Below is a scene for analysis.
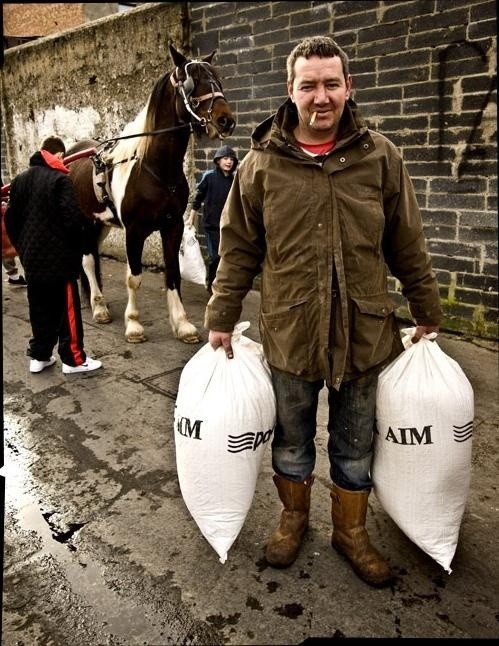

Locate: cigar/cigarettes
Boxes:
[309,111,317,126]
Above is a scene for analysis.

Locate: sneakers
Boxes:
[6,274,28,291]
[61,356,103,374]
[28,354,57,374]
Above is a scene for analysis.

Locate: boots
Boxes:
[264,472,317,569]
[329,478,395,590]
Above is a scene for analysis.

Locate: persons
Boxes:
[202,35,445,589]
[192,144,238,297]
[4,135,103,374]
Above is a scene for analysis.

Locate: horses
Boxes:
[62,42,238,346]
